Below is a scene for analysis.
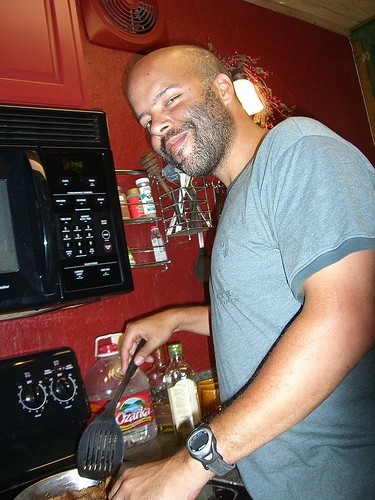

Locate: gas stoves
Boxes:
[0,346,92,500]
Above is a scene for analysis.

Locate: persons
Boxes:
[107,44,375,500]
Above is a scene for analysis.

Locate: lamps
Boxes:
[230,70,263,116]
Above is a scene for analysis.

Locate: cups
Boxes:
[197,377,221,418]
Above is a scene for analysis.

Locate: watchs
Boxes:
[185,422,236,477]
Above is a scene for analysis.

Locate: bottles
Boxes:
[135,178,157,215]
[126,188,145,219]
[117,186,131,221]
[84,332,158,449]
[165,344,204,440]
[151,226,168,262]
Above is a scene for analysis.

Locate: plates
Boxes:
[12,461,144,500]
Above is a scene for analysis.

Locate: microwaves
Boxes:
[0,102,135,321]
